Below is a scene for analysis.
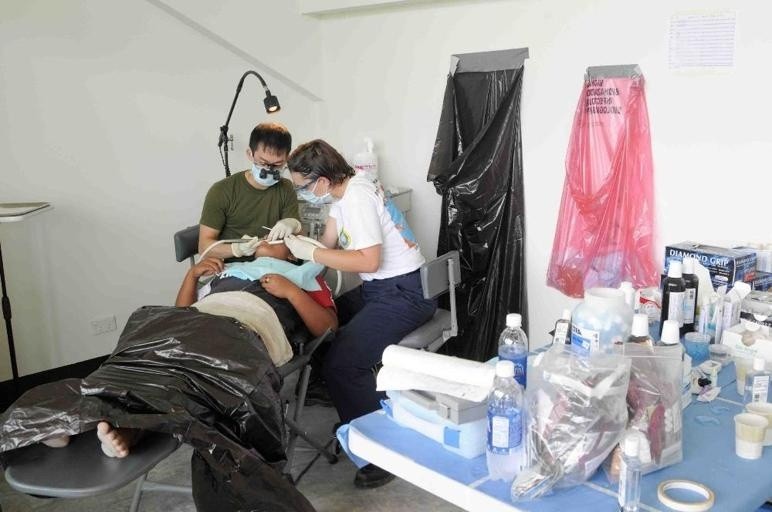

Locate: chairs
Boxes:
[170,220,214,292]
[6,235,343,512]
[393,250,462,354]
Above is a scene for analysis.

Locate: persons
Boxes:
[197,121,308,257]
[288,138,439,488]
[39,236,339,460]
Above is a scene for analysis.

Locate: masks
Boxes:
[300,177,332,206]
[250,157,286,187]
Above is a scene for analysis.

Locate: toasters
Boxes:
[552,310,571,344]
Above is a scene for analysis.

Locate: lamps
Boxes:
[220,68,281,176]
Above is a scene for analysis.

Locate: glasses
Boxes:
[252,154,286,172]
[293,178,319,194]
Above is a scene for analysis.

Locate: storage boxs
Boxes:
[664,242,756,284]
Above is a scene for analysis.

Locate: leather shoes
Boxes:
[353,463,395,489]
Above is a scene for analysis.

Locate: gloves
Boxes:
[284,234,316,265]
[268,218,302,241]
[231,234,262,258]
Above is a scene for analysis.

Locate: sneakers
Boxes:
[295,378,335,408]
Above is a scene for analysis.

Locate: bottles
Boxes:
[617,437,640,512]
[659,261,685,338]
[655,319,685,358]
[619,281,634,307]
[499,313,528,387]
[744,358,769,403]
[569,287,634,357]
[682,258,699,332]
[487,360,523,480]
[626,311,655,347]
[354,137,378,180]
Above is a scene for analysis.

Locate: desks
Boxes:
[0,202,50,387]
[343,287,772,511]
[297,187,412,240]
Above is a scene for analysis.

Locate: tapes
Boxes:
[657,478,714,512]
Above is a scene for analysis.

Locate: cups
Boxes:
[746,403,772,445]
[735,357,754,395]
[734,413,768,459]
[710,344,731,360]
[686,333,709,367]
